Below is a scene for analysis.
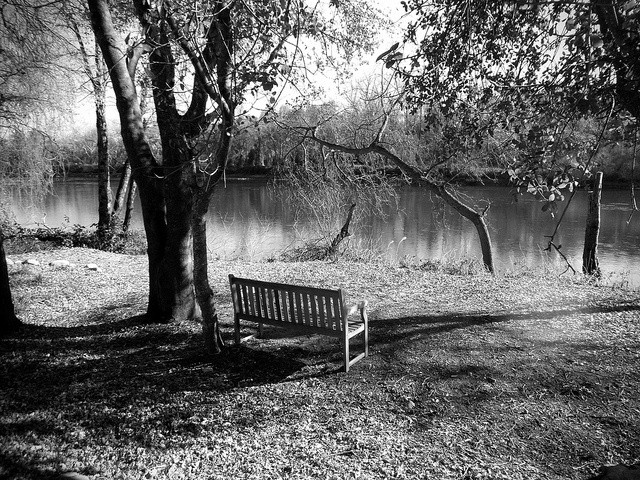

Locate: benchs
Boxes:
[226,273,370,373]
